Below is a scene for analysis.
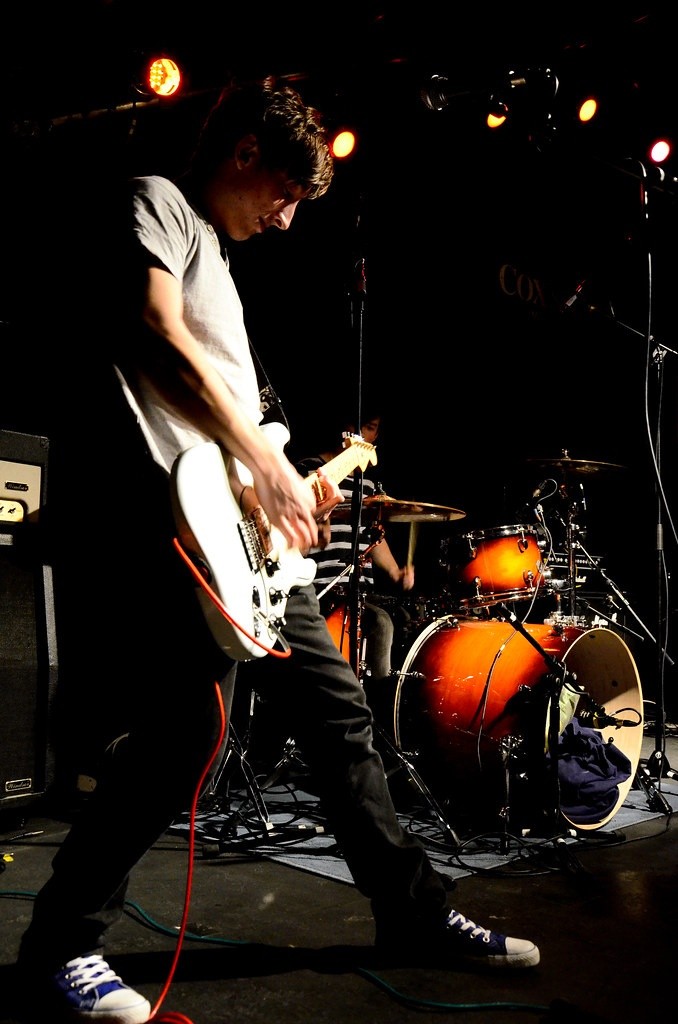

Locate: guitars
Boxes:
[167,419,381,665]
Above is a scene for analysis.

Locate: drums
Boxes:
[311,578,374,663]
[390,611,646,831]
[439,520,555,610]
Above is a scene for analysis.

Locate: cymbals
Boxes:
[346,493,469,528]
[525,456,619,474]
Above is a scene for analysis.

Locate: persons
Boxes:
[15,81,540,1024]
[298,401,415,679]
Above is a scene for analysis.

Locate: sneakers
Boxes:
[374,910,543,971]
[20,949,152,1024]
